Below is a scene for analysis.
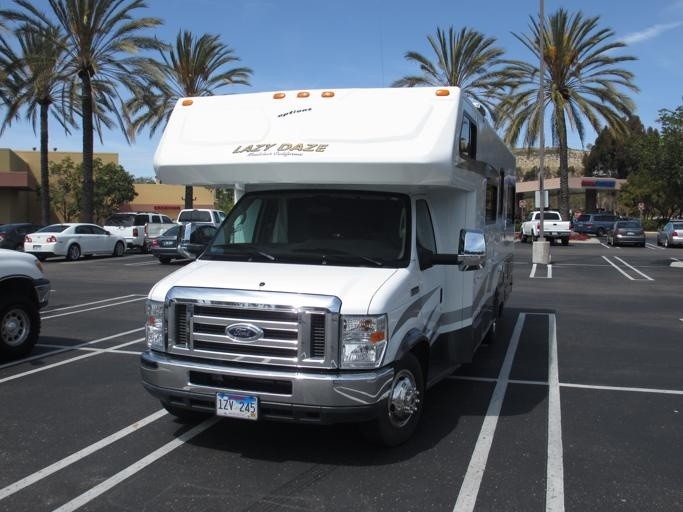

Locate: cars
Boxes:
[657,219,683,248]
[573,210,645,247]
[0,208,226,354]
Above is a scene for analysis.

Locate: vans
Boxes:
[140,86,517,443]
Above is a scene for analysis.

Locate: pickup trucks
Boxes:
[519,210,572,245]
